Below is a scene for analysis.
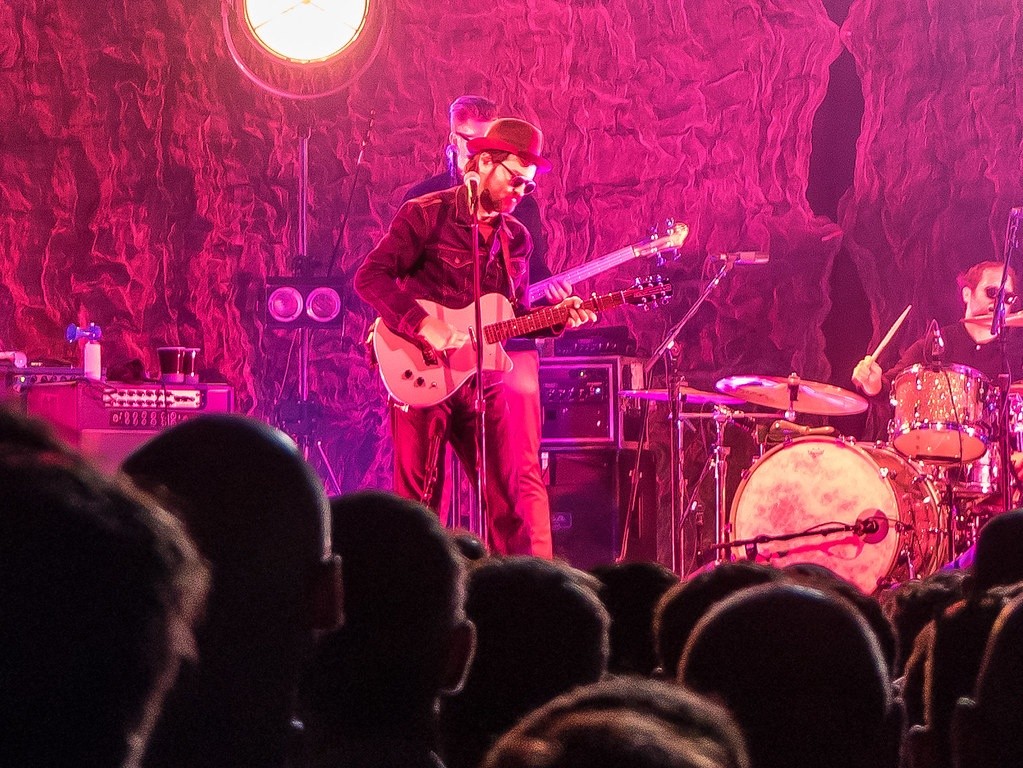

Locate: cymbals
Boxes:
[958,311,1023,327]
[716,373,869,416]
[619,388,748,404]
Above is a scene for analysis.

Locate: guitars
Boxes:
[371,274,676,407]
[528,216,691,304]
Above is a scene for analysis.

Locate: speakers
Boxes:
[533,447,656,573]
[21,380,234,478]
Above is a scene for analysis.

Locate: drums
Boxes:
[887,418,1005,499]
[730,434,950,599]
[891,360,995,464]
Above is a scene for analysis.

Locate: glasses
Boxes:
[493,159,536,195]
[984,287,1017,305]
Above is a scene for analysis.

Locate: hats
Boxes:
[466,118,553,175]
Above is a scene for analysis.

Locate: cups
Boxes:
[183,348,206,383]
[158,346,186,385]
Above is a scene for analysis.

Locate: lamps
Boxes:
[220,0,390,103]
[264,277,345,330]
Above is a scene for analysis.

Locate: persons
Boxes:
[353,119,597,559]
[0,402,1023,768]
[400,97,574,558]
[853,261,1023,404]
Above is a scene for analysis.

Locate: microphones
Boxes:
[852,520,880,534]
[464,171,481,213]
[710,402,756,431]
[932,323,941,373]
[712,251,770,264]
[1011,207,1023,218]
